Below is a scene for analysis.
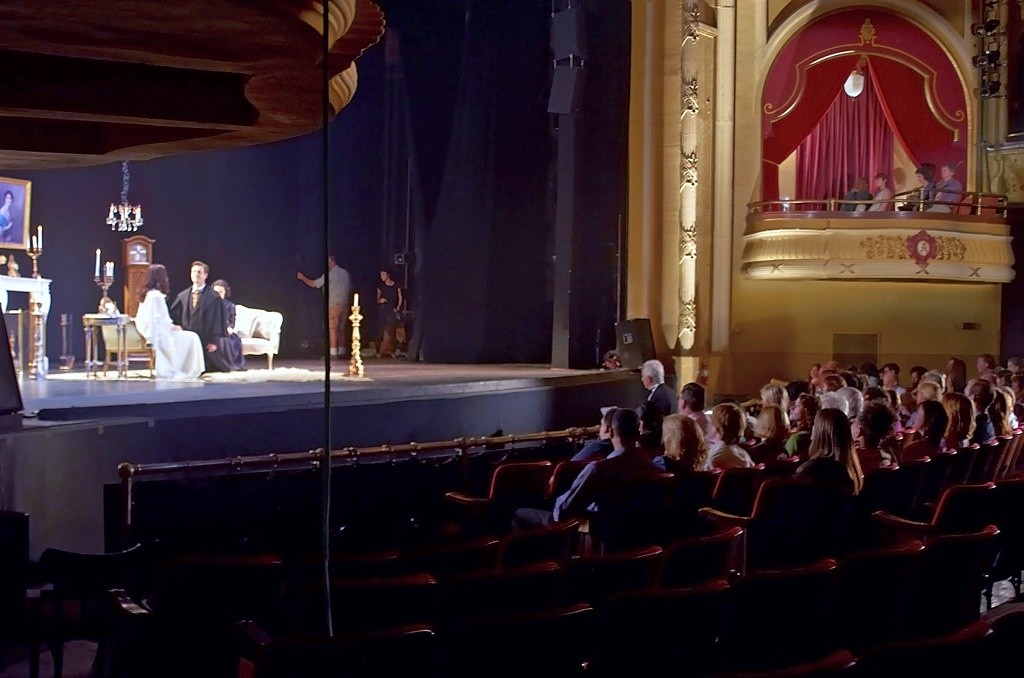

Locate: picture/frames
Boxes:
[0,176,32,249]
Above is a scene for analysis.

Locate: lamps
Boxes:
[843,70,865,102]
[26,225,43,278]
[94,248,114,314]
[971,18,1007,99]
[106,161,144,232]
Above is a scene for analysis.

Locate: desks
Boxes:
[1,275,53,380]
[83,313,130,379]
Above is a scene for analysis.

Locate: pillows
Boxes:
[233,305,259,338]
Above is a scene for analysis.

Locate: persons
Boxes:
[376,268,403,358]
[840,177,873,212]
[212,279,247,371]
[509,354,1024,529]
[297,254,352,360]
[915,167,935,199]
[135,265,206,378]
[927,163,962,212]
[169,261,230,372]
[867,173,891,211]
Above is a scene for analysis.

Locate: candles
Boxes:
[353,293,359,306]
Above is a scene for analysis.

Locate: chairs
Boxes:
[1,424,1024,677]
[102,317,156,379]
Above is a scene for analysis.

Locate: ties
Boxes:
[192,290,199,309]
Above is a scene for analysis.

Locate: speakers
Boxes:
[0,305,24,414]
[615,318,656,368]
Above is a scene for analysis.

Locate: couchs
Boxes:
[231,304,283,370]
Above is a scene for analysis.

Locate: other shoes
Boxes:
[321,354,338,360]
[338,354,346,359]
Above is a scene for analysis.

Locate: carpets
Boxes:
[46,367,375,384]
